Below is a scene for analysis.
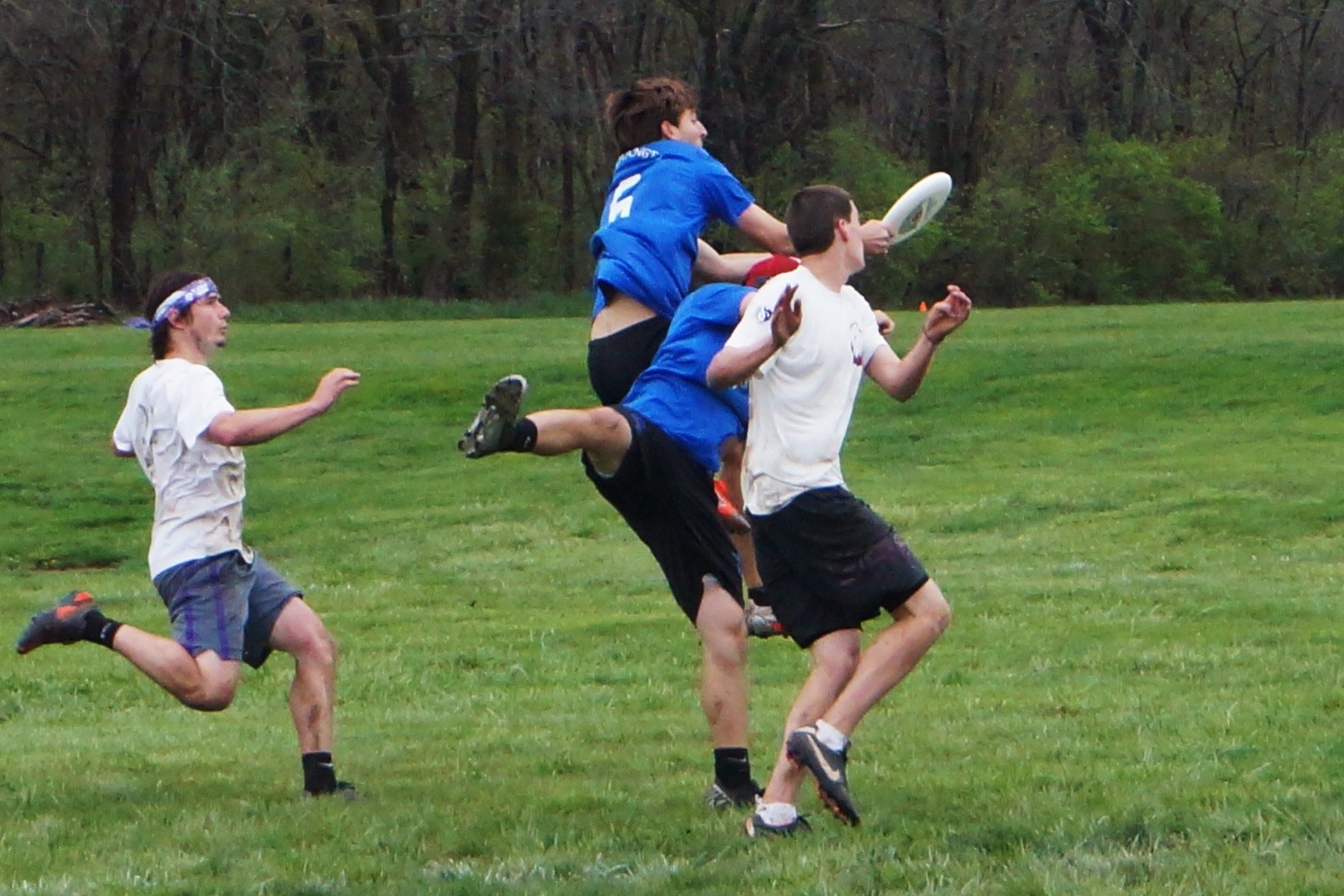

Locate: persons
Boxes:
[457,256,895,810]
[19,271,361,799]
[706,184,972,839]
[587,78,896,640]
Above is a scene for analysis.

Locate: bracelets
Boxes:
[922,325,943,343]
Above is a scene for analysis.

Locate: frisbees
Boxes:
[881,171,952,244]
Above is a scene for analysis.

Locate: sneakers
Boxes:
[711,780,764,814]
[713,484,755,535]
[14,589,99,655]
[304,782,365,800]
[742,598,790,638]
[743,812,809,836]
[458,373,527,460]
[786,725,861,827]
